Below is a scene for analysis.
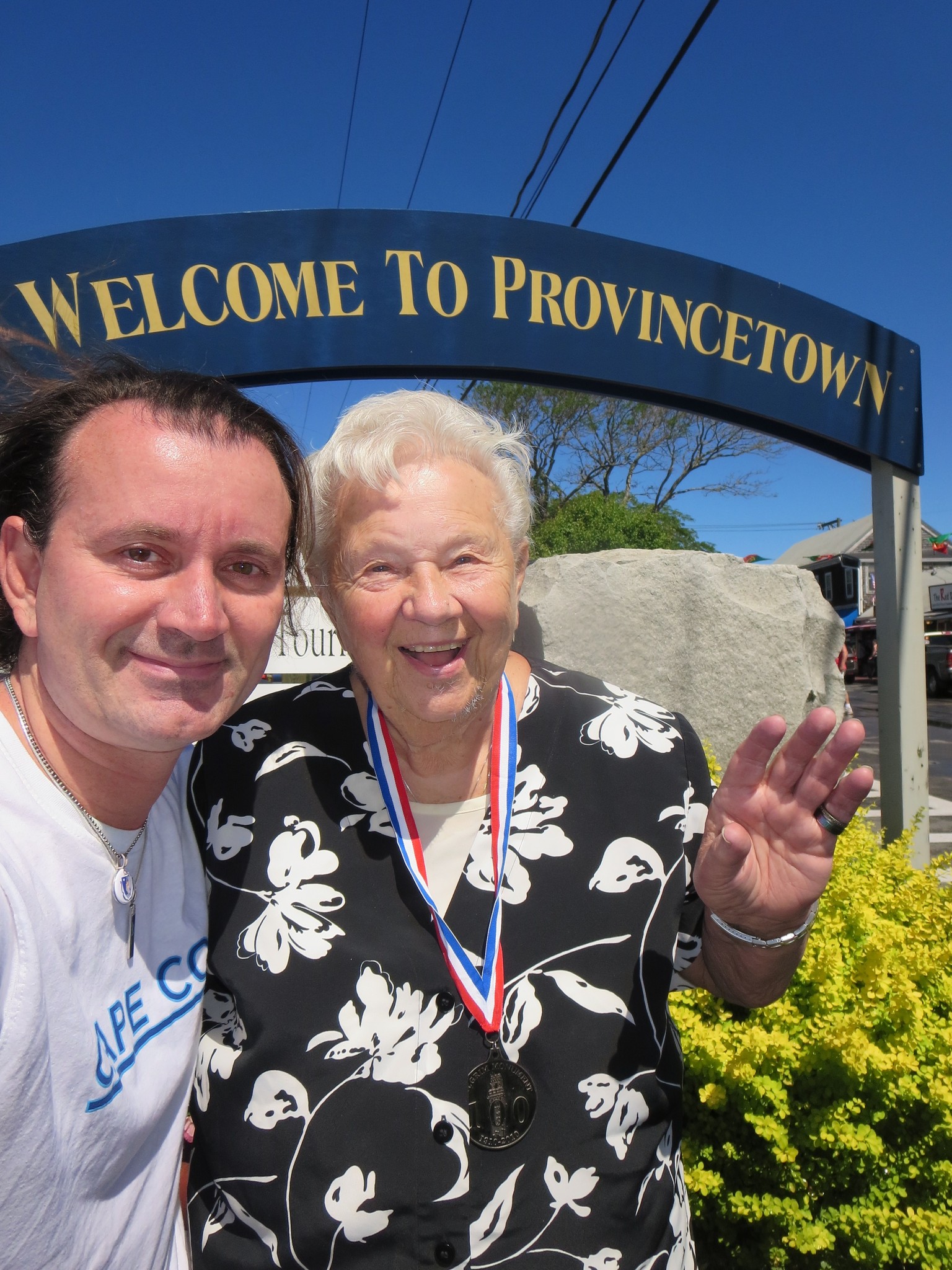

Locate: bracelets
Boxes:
[707,901,817,950]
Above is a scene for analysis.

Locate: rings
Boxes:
[816,804,847,836]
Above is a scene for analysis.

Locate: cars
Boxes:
[844,646,859,679]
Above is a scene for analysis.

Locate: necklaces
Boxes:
[4,673,150,959]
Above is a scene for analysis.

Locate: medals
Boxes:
[462,1056,539,1152]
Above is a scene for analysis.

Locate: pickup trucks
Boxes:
[925,645,952,695]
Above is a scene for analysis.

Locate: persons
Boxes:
[0,347,316,1269]
[183,390,876,1270]
[835,642,853,715]
[868,637,877,661]
